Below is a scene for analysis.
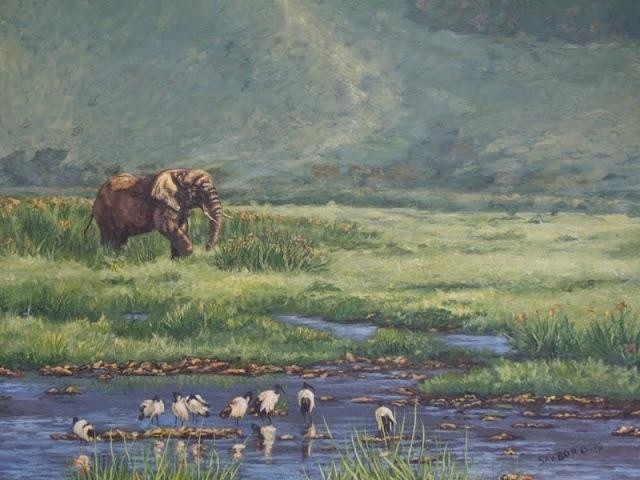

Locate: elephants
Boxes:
[82,167,235,262]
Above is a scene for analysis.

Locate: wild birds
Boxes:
[152,439,165,463]
[218,390,253,425]
[247,383,286,421]
[185,393,211,425]
[137,394,165,426]
[301,420,317,440]
[228,439,247,465]
[374,400,397,436]
[174,439,188,462]
[171,392,190,427]
[72,416,104,447]
[190,438,209,467]
[297,381,316,417]
[249,421,278,465]
[71,448,93,474]
